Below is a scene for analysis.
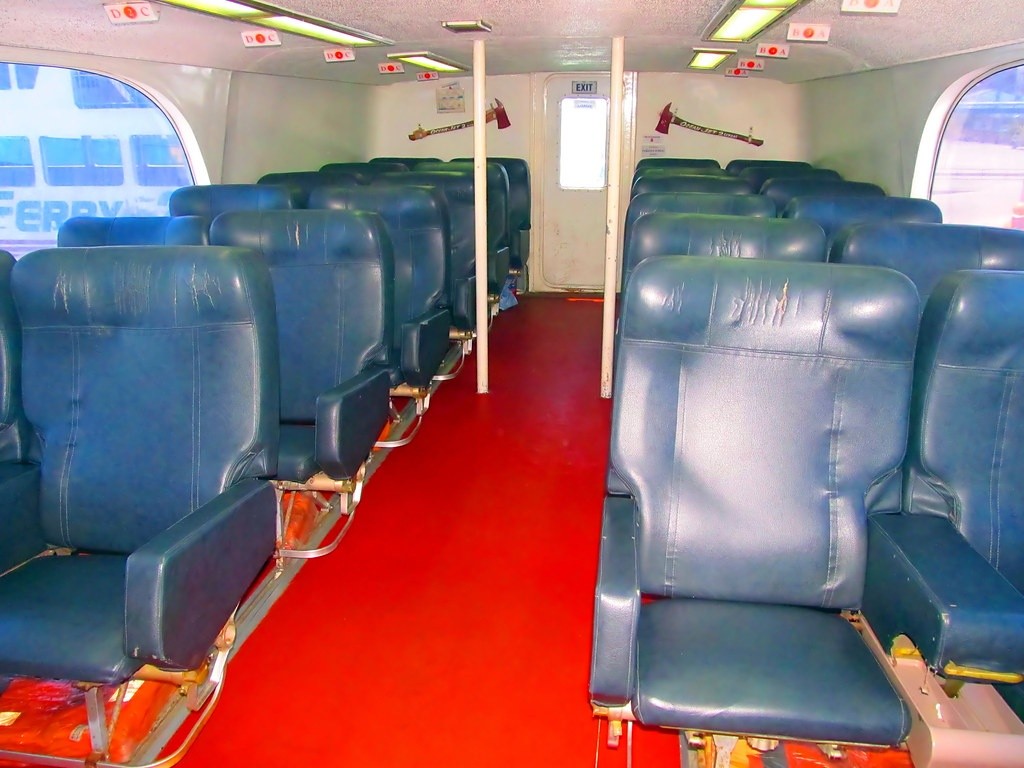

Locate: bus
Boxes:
[922,102,1023,229]
[1,63,194,260]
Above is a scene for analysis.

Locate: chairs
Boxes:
[0,158,531,768]
[590,158,1024,768]
